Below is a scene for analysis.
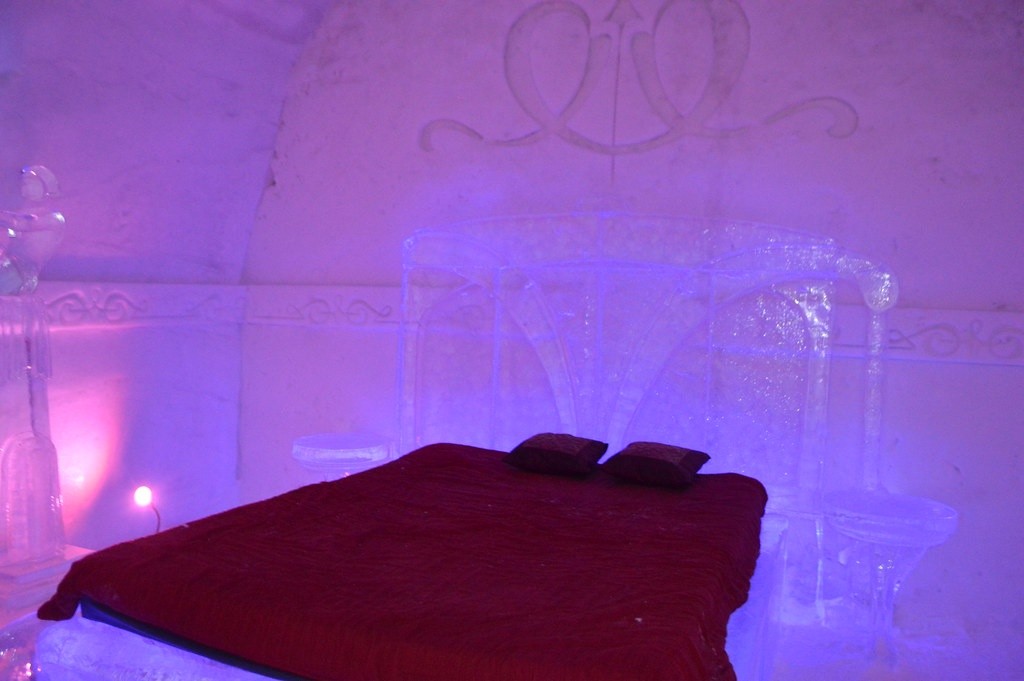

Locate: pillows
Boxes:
[502,432,610,480]
[600,441,710,491]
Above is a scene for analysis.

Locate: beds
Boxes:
[30,227,898,681]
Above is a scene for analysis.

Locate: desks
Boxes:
[820,493,956,664]
[292,433,388,481]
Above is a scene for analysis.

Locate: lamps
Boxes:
[131,485,162,535]
